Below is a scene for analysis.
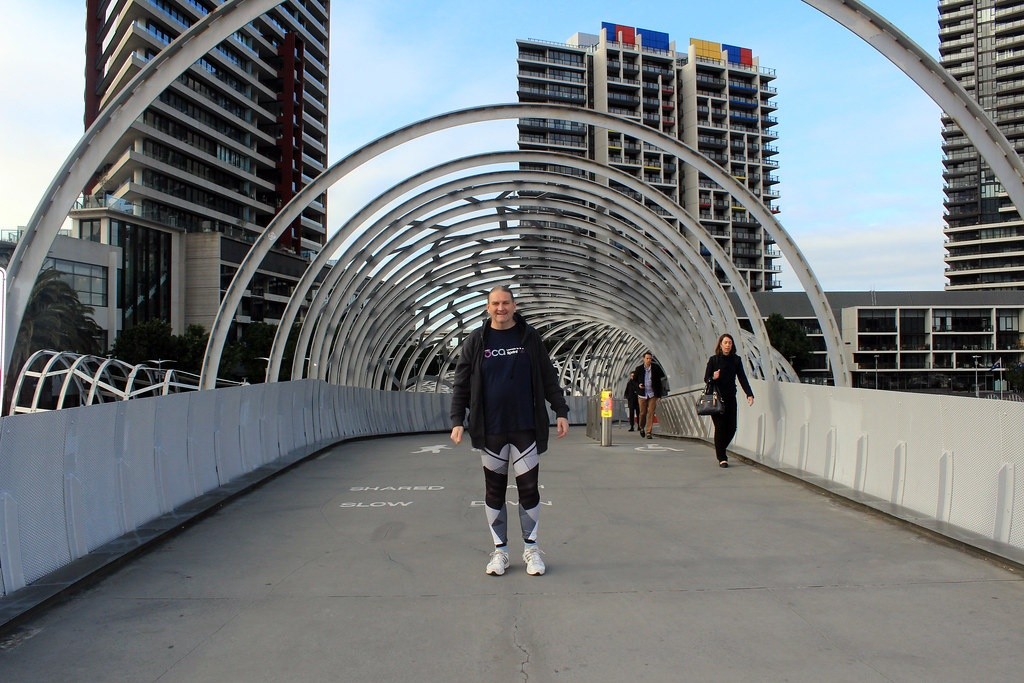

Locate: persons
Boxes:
[704,333,755,468]
[624,352,666,439]
[450,285,570,576]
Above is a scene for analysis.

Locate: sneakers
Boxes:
[719,458,728,468]
[646,432,652,439]
[486,549,510,576]
[628,428,634,431]
[522,545,546,576]
[640,428,645,437]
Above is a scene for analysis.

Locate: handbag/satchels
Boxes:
[696,375,724,415]
